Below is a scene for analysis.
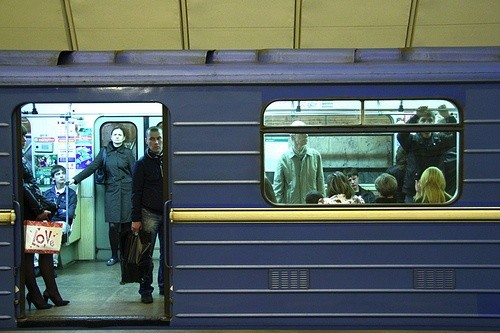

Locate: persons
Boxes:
[64,126,136,266]
[131,127,167,304]
[265,105,458,204]
[34,164,77,278]
[20,124,70,311]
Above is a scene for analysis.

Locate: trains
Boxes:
[0,46,500,331]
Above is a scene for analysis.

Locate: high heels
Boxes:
[26,292,53,311]
[43,290,70,306]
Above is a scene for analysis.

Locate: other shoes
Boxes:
[107,257,118,266]
[35,266,40,277]
[54,267,57,277]
[141,293,153,304]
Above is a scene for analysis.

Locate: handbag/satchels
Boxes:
[94,149,107,184]
[121,232,153,284]
[23,215,63,254]
